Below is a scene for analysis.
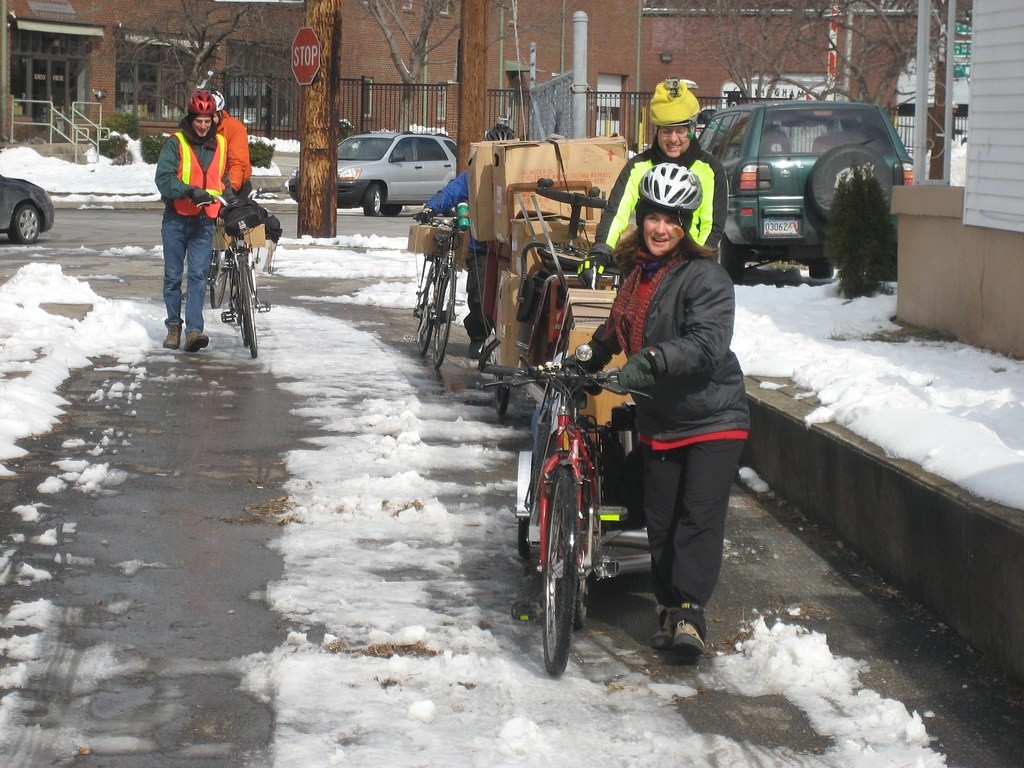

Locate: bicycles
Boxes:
[206,249,234,310]
[412,214,459,369]
[189,187,281,359]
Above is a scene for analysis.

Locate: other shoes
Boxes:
[469,340,483,358]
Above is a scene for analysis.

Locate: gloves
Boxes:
[188,185,210,207]
[618,346,667,394]
[577,253,609,292]
[415,207,434,225]
[564,338,614,408]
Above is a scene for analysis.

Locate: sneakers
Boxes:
[673,622,704,664]
[184,332,209,352]
[163,324,183,349]
[653,609,675,649]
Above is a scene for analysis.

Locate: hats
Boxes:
[637,196,693,240]
[648,77,701,125]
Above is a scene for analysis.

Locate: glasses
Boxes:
[658,127,688,138]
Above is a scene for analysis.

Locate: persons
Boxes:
[414,124,516,358]
[205,88,253,288]
[563,163,751,657]
[154,90,234,354]
[577,78,728,287]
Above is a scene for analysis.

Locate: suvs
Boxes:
[288,131,458,217]
[698,100,913,284]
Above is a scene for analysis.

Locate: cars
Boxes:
[0,174,55,244]
[695,109,717,139]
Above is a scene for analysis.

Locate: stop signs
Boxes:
[291,27,321,85]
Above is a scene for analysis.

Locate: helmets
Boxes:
[485,124,514,142]
[210,89,225,111]
[636,163,704,213]
[188,89,216,115]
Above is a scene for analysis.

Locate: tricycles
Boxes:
[493,268,622,414]
[481,343,652,677]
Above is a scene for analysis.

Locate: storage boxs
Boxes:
[468,142,524,242]
[561,289,637,426]
[408,226,471,254]
[209,220,266,250]
[491,137,629,242]
[497,270,529,366]
[507,209,598,279]
